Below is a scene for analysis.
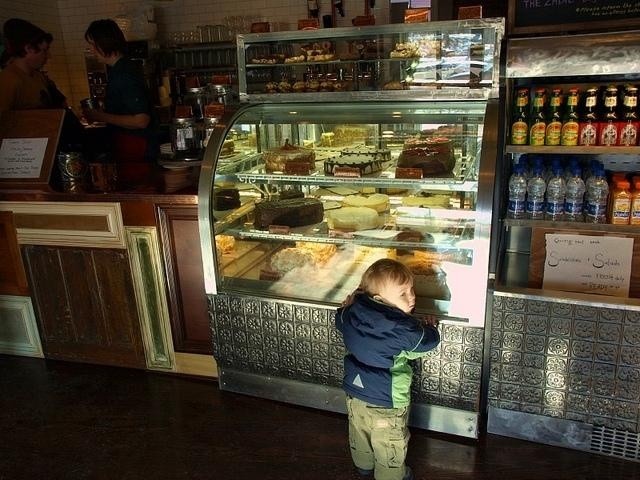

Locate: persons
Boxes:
[0,19,84,153]
[0,16,52,111]
[84,18,161,192]
[335,258,440,479]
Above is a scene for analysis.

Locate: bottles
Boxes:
[205,84,232,124]
[172,118,198,157]
[81,98,101,110]
[562,89,579,146]
[566,170,585,222]
[528,167,545,219]
[203,117,219,152]
[610,181,631,225]
[546,168,566,221]
[599,88,620,146]
[611,171,640,191]
[631,181,640,225]
[177,87,203,119]
[511,89,528,145]
[620,87,640,146]
[508,168,525,219]
[585,170,609,223]
[513,154,605,178]
[579,89,599,146]
[529,89,546,145]
[546,90,562,145]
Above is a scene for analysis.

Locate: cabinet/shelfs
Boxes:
[0,162,221,382]
[84,34,384,123]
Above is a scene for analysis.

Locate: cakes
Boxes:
[264,142,317,173]
[223,138,235,156]
[396,136,456,176]
[297,242,337,265]
[344,193,389,214]
[215,235,235,253]
[325,154,382,175]
[330,207,380,232]
[420,125,473,146]
[403,192,450,209]
[270,247,320,278]
[213,185,242,211]
[320,125,372,148]
[343,146,391,161]
[386,247,452,301]
[255,197,323,231]
[393,206,436,232]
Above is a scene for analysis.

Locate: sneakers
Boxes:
[356,465,373,476]
[402,465,414,479]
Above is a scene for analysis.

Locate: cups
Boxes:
[245,42,294,60]
[59,151,87,193]
[89,163,118,192]
[194,25,205,43]
[215,25,225,42]
[205,25,215,42]
[170,30,195,43]
[170,48,237,67]
[322,15,333,28]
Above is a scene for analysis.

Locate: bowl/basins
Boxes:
[250,21,270,33]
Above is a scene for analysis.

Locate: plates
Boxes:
[164,164,190,172]
[159,143,174,155]
